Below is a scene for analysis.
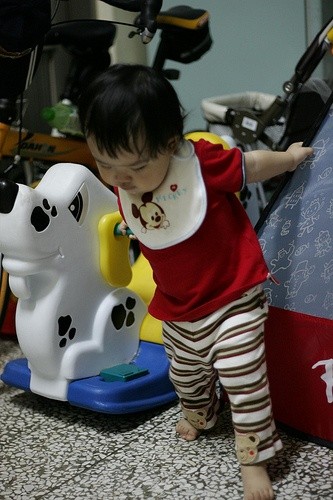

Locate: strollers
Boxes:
[200,12,333,208]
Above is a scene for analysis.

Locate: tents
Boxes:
[253,89,333,450]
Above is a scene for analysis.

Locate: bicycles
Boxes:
[0,0,214,333]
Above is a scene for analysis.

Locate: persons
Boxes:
[77,62,313,500]
